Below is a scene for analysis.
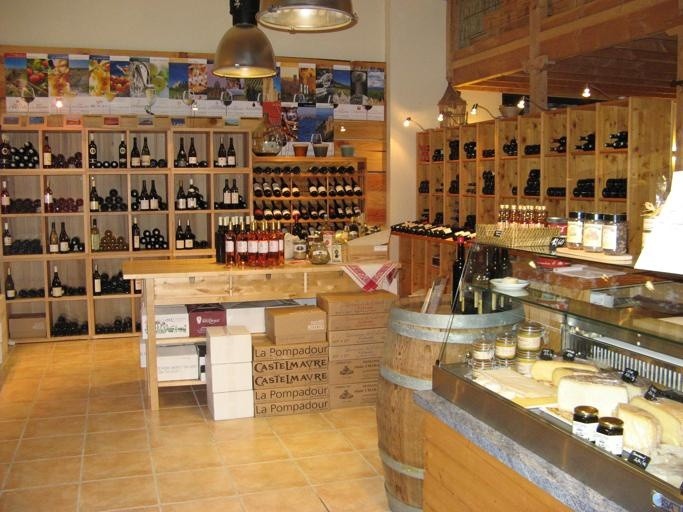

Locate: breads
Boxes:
[606,371,653,395]
[531,352,598,382]
[576,282,682,316]
[631,392,683,450]
[632,314,682,342]
[555,371,629,420]
[615,404,661,456]
[566,299,634,327]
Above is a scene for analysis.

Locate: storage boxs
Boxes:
[140,289,398,421]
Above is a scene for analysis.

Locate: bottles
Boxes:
[454,237,514,311]
[498,203,549,227]
[567,211,628,256]
[0,174,248,213]
[470,320,544,380]
[251,111,284,157]
[420,206,476,226]
[412,165,628,199]
[573,406,625,458]
[6,257,141,338]
[1,131,238,168]
[292,235,330,264]
[216,215,287,266]
[2,214,211,251]
[391,219,477,241]
[429,127,633,161]
[252,165,370,239]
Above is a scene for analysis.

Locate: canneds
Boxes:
[572,405,596,443]
[517,321,544,352]
[517,353,537,374]
[494,362,517,374]
[292,240,306,259]
[596,418,624,458]
[494,336,517,361]
[473,363,495,380]
[306,234,325,257]
[471,338,494,360]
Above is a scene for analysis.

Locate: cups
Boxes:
[293,143,308,157]
[294,94,305,107]
[314,144,328,158]
[310,133,323,147]
[342,145,355,157]
[327,94,340,109]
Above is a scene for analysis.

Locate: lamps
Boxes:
[211,0,277,78]
[582,82,613,100]
[255,0,359,34]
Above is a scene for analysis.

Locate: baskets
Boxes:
[475,223,562,249]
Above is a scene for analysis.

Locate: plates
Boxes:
[491,277,531,290]
[497,289,531,297]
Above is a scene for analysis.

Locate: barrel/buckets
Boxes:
[375,292,528,512]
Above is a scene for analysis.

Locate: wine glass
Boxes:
[21,88,37,113]
[183,90,195,116]
[62,84,78,113]
[361,93,373,120]
[104,90,117,114]
[145,88,157,115]
[220,90,233,117]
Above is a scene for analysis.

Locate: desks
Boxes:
[121,258,401,410]
